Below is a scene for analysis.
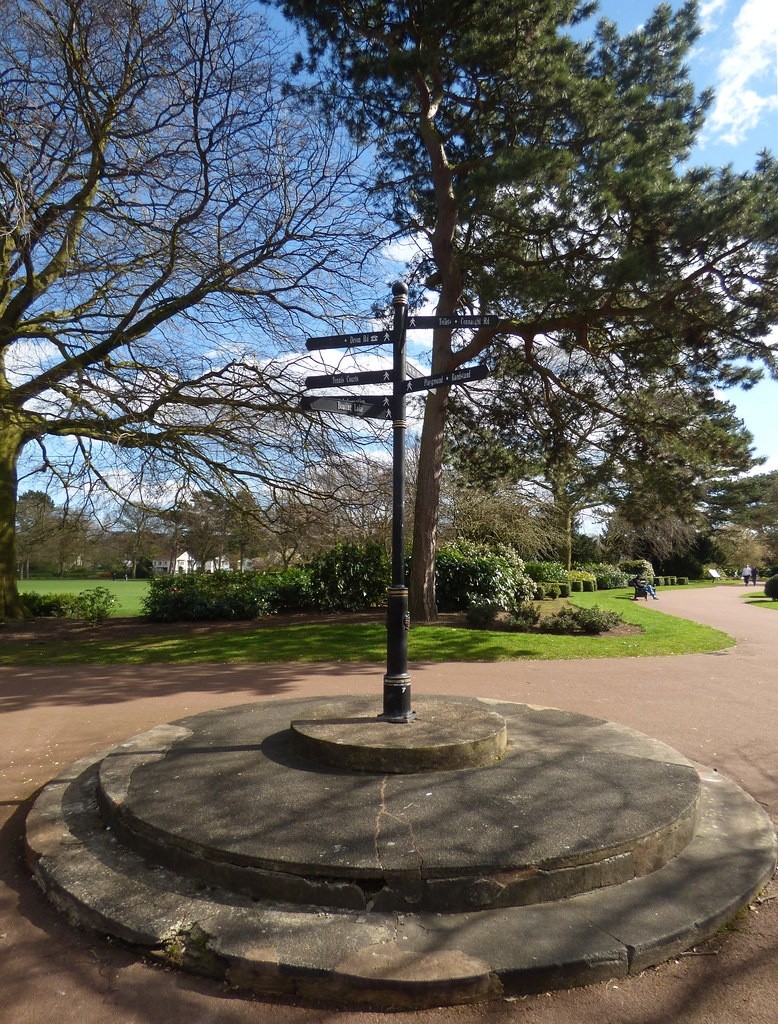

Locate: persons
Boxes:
[751,567,759,587]
[635,575,659,600]
[742,564,752,586]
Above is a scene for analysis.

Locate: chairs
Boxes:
[631,578,648,601]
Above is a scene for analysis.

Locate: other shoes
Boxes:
[653,596,660,600]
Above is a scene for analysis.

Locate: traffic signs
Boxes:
[304,329,394,351]
[407,364,491,393]
[307,395,393,420]
[299,394,392,412]
[406,314,500,330]
[304,368,394,389]
